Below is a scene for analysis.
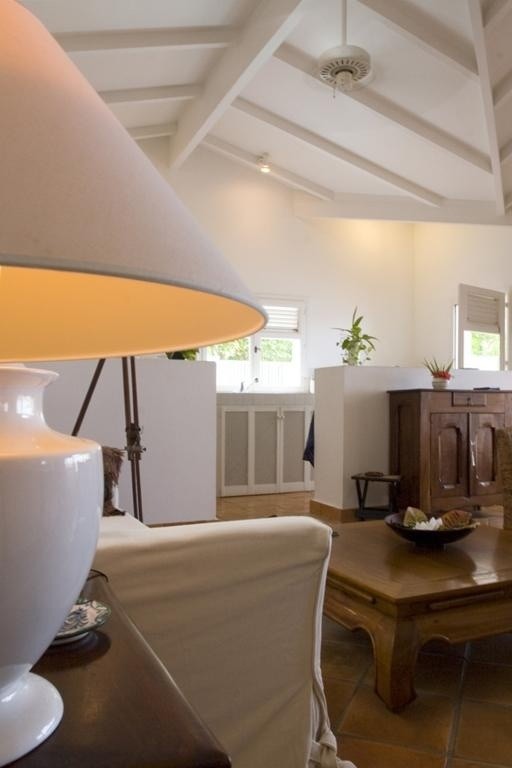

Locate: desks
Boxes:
[324,519,511,714]
[350,473,404,521]
[13,567,232,766]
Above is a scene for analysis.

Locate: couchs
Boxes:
[94,445,333,767]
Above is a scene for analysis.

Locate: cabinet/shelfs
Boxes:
[385,389,511,516]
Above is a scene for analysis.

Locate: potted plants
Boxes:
[333,307,376,366]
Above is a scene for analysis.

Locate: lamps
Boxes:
[315,0,372,95]
[0,0,269,766]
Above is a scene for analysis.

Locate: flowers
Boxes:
[424,355,457,380]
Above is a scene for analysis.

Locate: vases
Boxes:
[431,377,449,390]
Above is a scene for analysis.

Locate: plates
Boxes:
[46,598,112,646]
[384,511,480,551]
